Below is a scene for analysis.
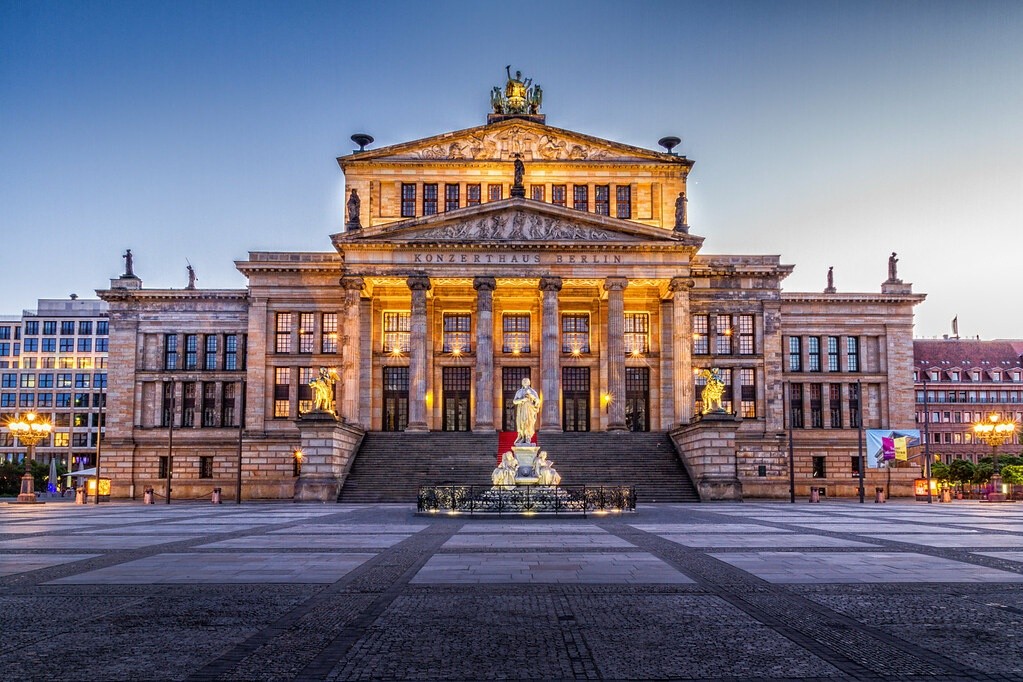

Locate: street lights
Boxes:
[971,411,1017,501]
[8,410,54,503]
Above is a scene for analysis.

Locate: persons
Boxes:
[674,192,689,227]
[514,153,526,188]
[122,249,134,275]
[347,188,361,224]
[313,369,332,404]
[512,377,541,443]
[492,451,518,492]
[186,265,195,287]
[827,266,834,288]
[889,252,899,278]
[706,367,724,409]
[534,451,561,488]
